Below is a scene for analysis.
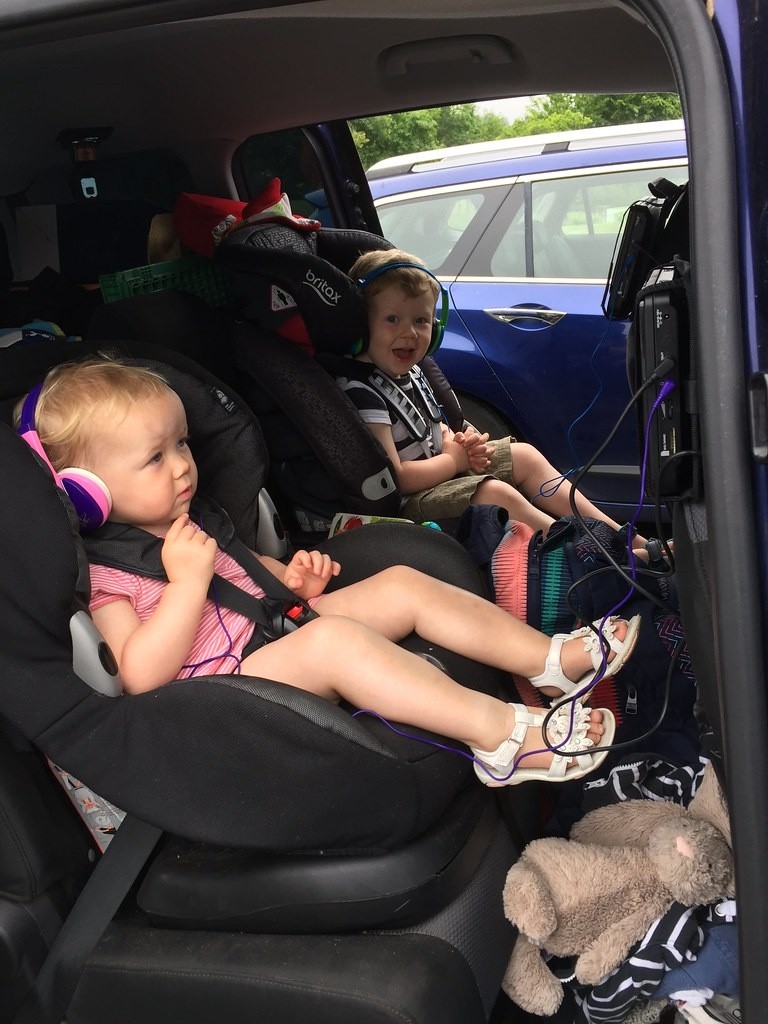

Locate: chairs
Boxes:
[503,221,585,279]
[87,293,242,392]
[0,340,519,1024]
[210,223,517,588]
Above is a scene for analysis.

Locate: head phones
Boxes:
[350,261,449,359]
[19,381,112,532]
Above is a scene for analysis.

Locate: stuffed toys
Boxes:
[501,758,734,1016]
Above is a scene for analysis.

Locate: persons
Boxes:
[12,359,640,787]
[331,248,676,568]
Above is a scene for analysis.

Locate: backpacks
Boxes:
[463,503,697,766]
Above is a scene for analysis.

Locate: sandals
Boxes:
[528,614,642,708]
[473,702,616,787]
[645,538,673,572]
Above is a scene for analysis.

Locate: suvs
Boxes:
[303,118,690,538]
